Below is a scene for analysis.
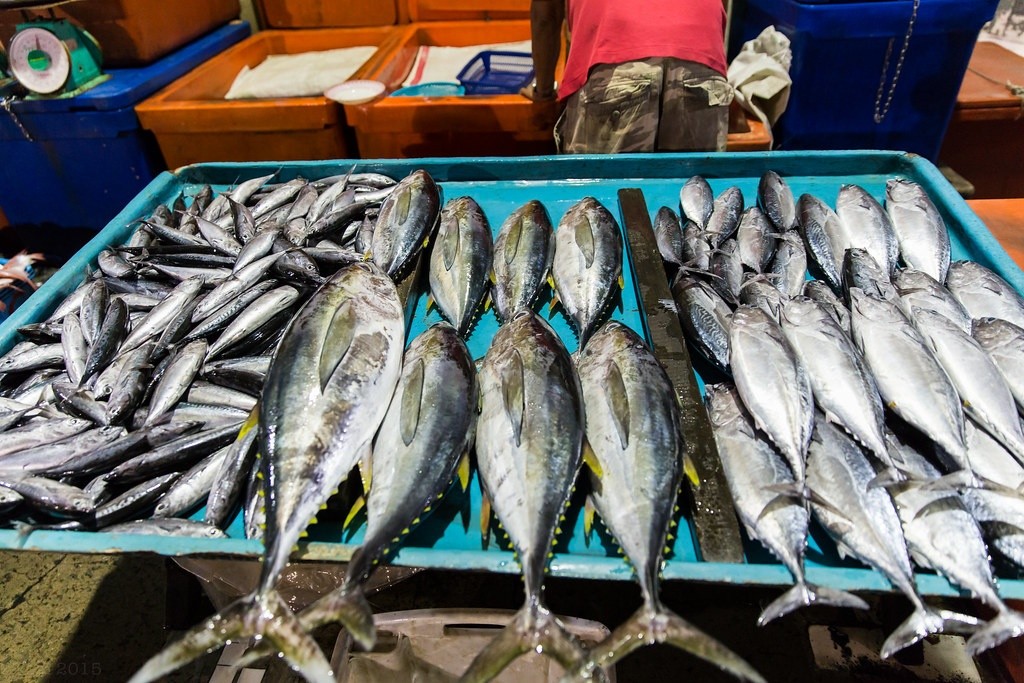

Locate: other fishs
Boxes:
[0,161,1024,683]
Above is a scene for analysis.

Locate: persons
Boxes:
[553,0,735,155]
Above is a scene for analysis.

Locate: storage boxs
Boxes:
[251,0,409,28]
[134,25,404,169]
[343,16,567,160]
[728,0,1000,165]
[406,0,530,23]
[0,0,242,68]
[0,20,252,228]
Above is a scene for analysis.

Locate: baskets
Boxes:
[456,49,535,93]
[387,81,466,98]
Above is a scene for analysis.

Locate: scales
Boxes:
[7,0,113,102]
